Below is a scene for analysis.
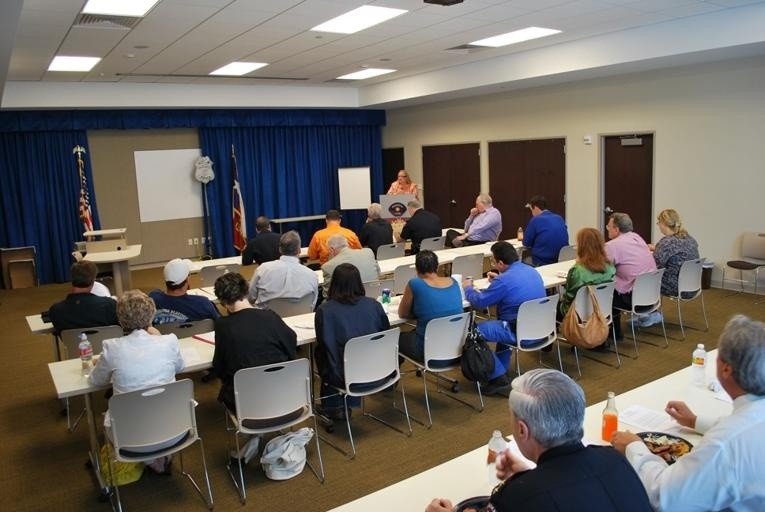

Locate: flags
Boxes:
[230,155,251,251]
[77,159,98,239]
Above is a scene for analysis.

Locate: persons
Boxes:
[422,366,653,512]
[607,309,765,511]
[320,230,382,304]
[144,255,224,323]
[313,259,390,421]
[598,214,656,337]
[521,196,566,266]
[382,168,416,194]
[443,190,502,250]
[398,246,461,366]
[85,284,187,479]
[648,208,701,302]
[50,261,120,342]
[393,199,441,253]
[554,228,616,321]
[240,215,288,264]
[245,231,320,312]
[463,240,546,393]
[207,269,305,431]
[306,210,363,265]
[355,203,393,251]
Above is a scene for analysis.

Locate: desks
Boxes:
[320,349,734,511]
[41,260,579,499]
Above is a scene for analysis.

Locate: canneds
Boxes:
[467,277,473,285]
[381,288,391,304]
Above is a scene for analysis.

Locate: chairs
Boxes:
[495,292,563,378]
[311,327,412,461]
[670,256,709,341]
[105,379,216,510]
[393,311,486,430]
[58,323,126,434]
[720,232,765,305]
[25,228,581,362]
[225,359,327,507]
[614,268,670,359]
[557,282,622,377]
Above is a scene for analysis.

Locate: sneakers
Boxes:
[626,312,661,327]
[452,240,464,248]
[316,404,352,420]
[477,376,513,395]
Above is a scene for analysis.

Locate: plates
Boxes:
[635,432,693,465]
[448,494,493,512]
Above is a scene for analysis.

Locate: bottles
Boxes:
[485,429,508,489]
[517,227,525,241]
[693,343,707,385]
[602,390,618,444]
[78,331,94,378]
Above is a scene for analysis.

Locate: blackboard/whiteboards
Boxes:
[336,165,373,210]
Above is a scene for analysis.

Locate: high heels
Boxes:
[230,437,258,464]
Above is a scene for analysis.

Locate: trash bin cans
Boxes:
[701,262,714,289]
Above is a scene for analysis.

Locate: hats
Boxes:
[163,258,193,286]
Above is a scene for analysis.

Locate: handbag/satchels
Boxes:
[560,310,610,349]
[100,443,144,487]
[462,332,495,381]
[260,427,314,480]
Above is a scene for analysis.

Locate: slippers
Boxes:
[164,456,172,476]
